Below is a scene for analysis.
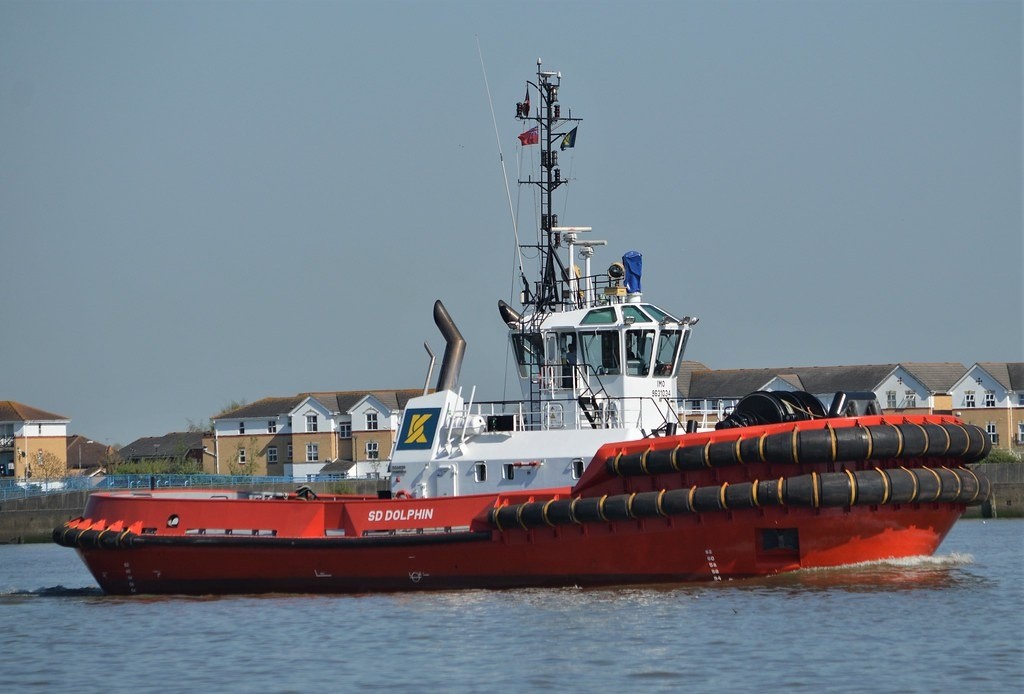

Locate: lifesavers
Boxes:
[662,364,673,376]
[394,489,411,499]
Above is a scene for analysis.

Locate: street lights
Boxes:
[77,440,94,474]
[351,435,358,479]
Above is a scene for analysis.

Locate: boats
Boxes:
[52,32,992,596]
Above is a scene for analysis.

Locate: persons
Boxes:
[561,344,576,363]
[627,346,635,359]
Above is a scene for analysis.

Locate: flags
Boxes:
[524,90,530,117]
[560,126,578,150]
[518,126,538,145]
